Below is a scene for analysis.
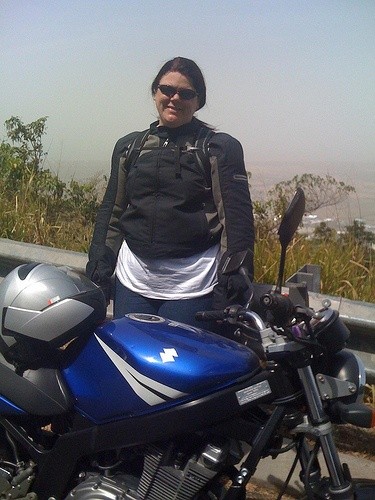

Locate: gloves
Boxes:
[212,272,252,307]
[86,261,112,305]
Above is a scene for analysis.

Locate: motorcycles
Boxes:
[0,186,375,500]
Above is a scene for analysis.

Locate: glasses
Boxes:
[156,85,199,100]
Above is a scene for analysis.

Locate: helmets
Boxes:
[0,262,106,369]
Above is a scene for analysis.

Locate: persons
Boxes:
[83,56,255,329]
